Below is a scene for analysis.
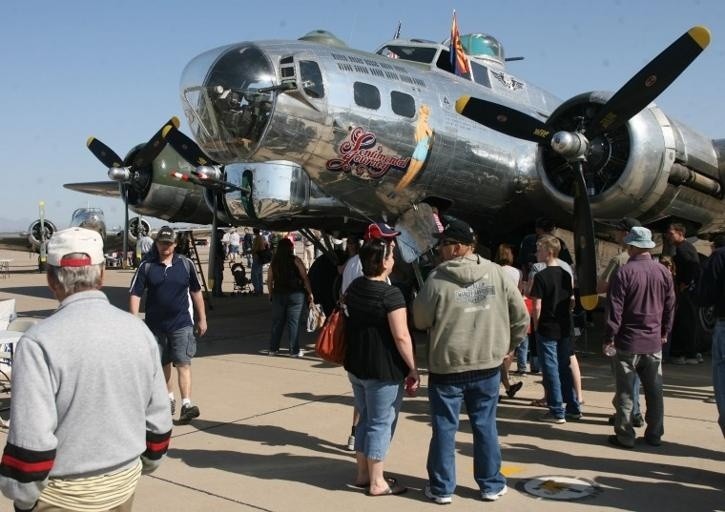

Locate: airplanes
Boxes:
[59,11,724,300]
[0,201,229,271]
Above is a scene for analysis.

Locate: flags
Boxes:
[387,22,402,58]
[449,12,470,76]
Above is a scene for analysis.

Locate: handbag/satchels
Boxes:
[305,299,327,333]
[315,292,350,364]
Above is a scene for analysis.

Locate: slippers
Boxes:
[366,483,408,496]
[356,477,397,487]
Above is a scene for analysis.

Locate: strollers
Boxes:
[230,262,254,297]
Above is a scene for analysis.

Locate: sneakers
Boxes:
[425,478,452,504]
[346,435,356,449]
[171,397,177,415]
[290,350,304,358]
[674,355,699,365]
[179,402,200,425]
[506,382,522,397]
[532,395,661,450]
[480,483,507,501]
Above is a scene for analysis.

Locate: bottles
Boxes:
[602,344,617,357]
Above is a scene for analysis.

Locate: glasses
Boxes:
[436,240,454,246]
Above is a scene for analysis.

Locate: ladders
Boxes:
[177,230,213,310]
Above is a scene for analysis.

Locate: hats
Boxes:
[157,226,176,243]
[46,227,107,269]
[433,218,478,245]
[622,225,656,249]
[363,221,401,242]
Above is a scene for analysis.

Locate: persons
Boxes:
[0,229,173,512]
[127,226,208,422]
[138,233,154,261]
